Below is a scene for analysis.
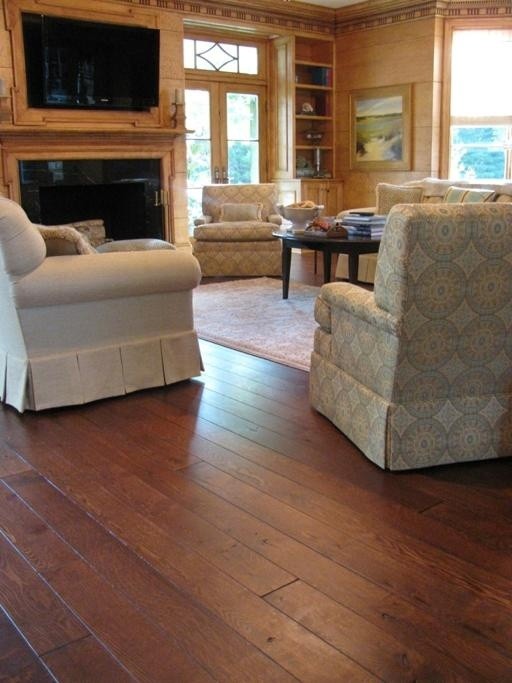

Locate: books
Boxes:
[340,215,386,236]
[316,91,333,117]
[313,69,332,87]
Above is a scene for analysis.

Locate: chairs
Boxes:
[192,183,283,277]
[0,196,205,413]
[308,203,512,471]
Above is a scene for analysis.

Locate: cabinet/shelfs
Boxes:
[268,33,344,179]
[0,0,194,254]
[270,179,343,254]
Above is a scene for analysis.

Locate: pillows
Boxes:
[95,238,176,253]
[219,203,263,223]
[443,186,496,203]
[34,224,98,256]
[375,183,424,217]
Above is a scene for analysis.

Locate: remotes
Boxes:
[350,211,374,216]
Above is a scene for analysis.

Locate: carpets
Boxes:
[192,277,321,373]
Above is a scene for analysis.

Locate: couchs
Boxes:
[335,177,512,284]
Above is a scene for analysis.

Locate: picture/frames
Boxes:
[348,83,414,172]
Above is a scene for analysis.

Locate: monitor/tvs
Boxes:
[21,9,159,111]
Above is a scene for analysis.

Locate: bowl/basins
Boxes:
[277,203,325,232]
